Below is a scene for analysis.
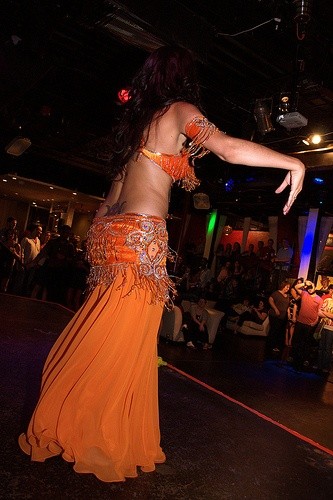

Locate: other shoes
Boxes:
[203,343,213,350]
[186,341,196,350]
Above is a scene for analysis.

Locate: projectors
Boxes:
[276,111,308,130]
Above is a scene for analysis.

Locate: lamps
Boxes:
[291,0,312,41]
[252,98,275,134]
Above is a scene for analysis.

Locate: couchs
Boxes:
[219,307,271,346]
[160,296,226,344]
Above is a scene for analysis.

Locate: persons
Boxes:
[0,218,93,311]
[163,238,333,372]
[18,43,306,482]
